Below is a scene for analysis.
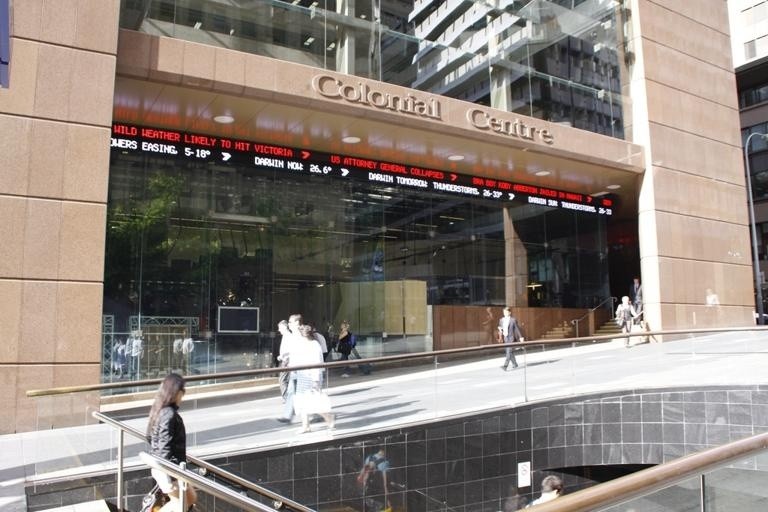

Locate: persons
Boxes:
[326,320,338,348]
[525,475,564,509]
[338,320,352,377]
[482,307,495,344]
[615,295,638,347]
[628,277,643,324]
[357,443,392,512]
[277,314,336,434]
[705,288,723,314]
[113,329,194,380]
[145,374,197,512]
[498,307,524,370]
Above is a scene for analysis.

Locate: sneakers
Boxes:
[341,374,352,378]
[277,411,337,434]
[500,365,518,372]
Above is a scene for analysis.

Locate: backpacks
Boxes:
[363,456,388,488]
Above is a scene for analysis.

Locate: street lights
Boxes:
[745,132,768,325]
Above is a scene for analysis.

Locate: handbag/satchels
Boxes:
[291,391,332,417]
[615,310,624,326]
[140,483,194,512]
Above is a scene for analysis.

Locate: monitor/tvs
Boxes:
[217,306,259,333]
